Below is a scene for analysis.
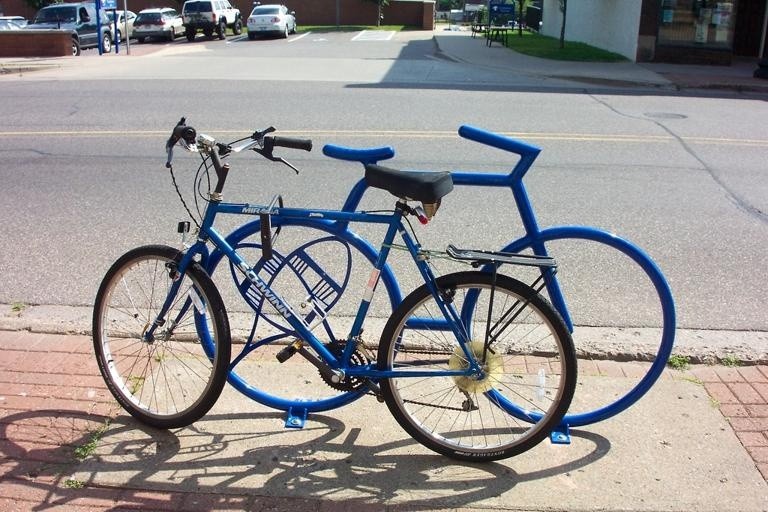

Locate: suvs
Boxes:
[182,0,243,41]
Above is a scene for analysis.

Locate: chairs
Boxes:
[470,12,509,49]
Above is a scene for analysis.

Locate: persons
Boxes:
[462,10,482,28]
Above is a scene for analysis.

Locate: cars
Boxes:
[247,5,297,40]
[0,0,186,56]
